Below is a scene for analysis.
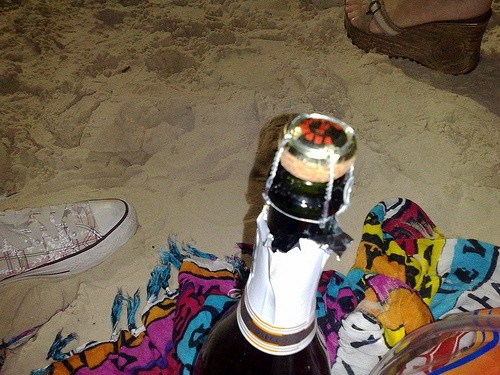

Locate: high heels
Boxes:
[344,0,492,75]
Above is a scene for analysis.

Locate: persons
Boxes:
[344,0,494,76]
[1,197,138,286]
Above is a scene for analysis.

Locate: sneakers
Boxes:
[0,198,138,286]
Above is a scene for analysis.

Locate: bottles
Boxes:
[191,111,357,375]
